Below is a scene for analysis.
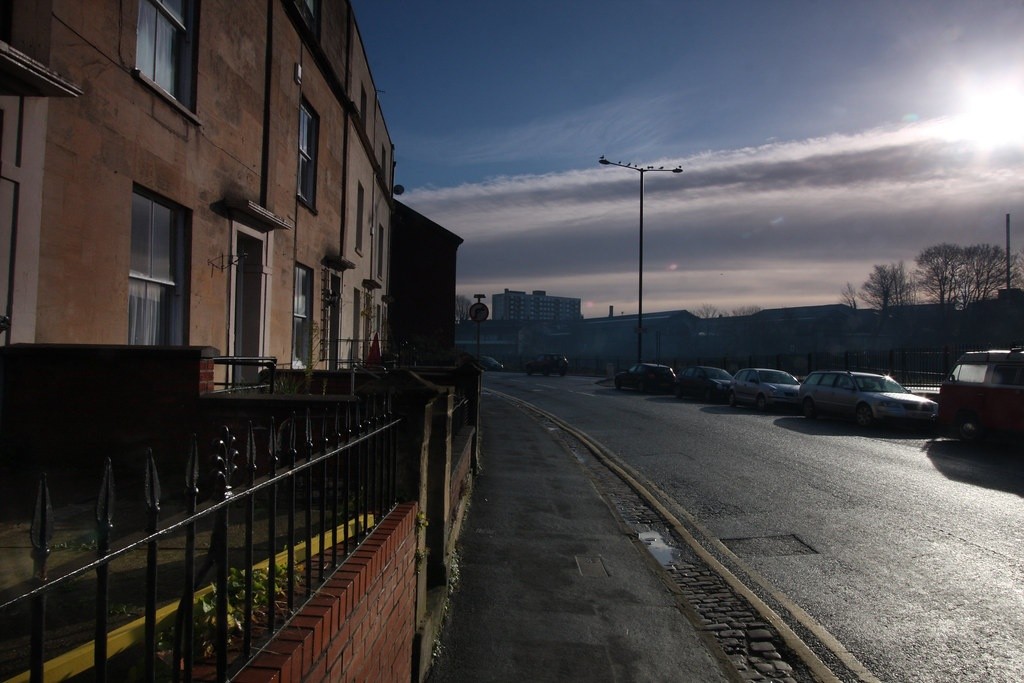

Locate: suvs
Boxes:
[938,345,1024,446]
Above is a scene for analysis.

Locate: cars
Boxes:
[454,349,504,373]
[677,365,733,404]
[614,362,678,396]
[525,353,569,378]
[728,367,802,415]
[799,370,939,430]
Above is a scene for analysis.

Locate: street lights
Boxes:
[599,158,683,363]
